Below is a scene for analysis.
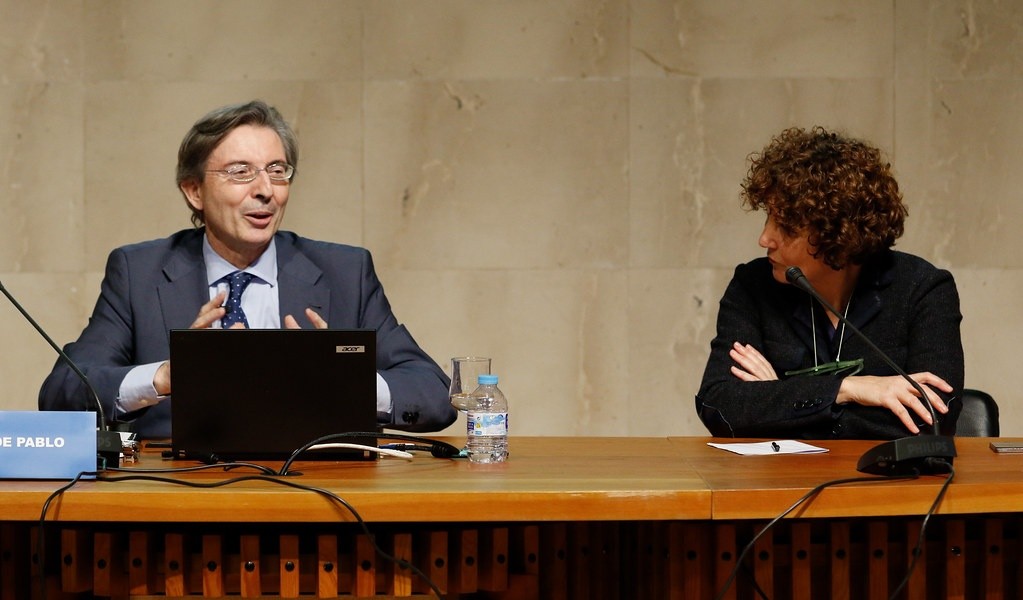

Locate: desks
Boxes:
[0,436,1023,600]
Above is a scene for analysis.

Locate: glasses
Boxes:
[785,358,864,377]
[205,163,294,183]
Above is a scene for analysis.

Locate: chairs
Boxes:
[954,389,1000,438]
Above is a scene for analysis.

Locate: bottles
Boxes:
[466,374,509,464]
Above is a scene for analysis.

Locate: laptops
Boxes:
[169,328,379,462]
[781,265,957,477]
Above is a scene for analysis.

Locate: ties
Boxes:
[222,272,257,329]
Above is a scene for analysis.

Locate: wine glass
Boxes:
[448,356,491,458]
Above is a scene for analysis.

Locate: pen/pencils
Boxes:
[771,442,780,452]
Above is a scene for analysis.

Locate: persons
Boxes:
[38,99,458,441]
[695,132,965,439]
